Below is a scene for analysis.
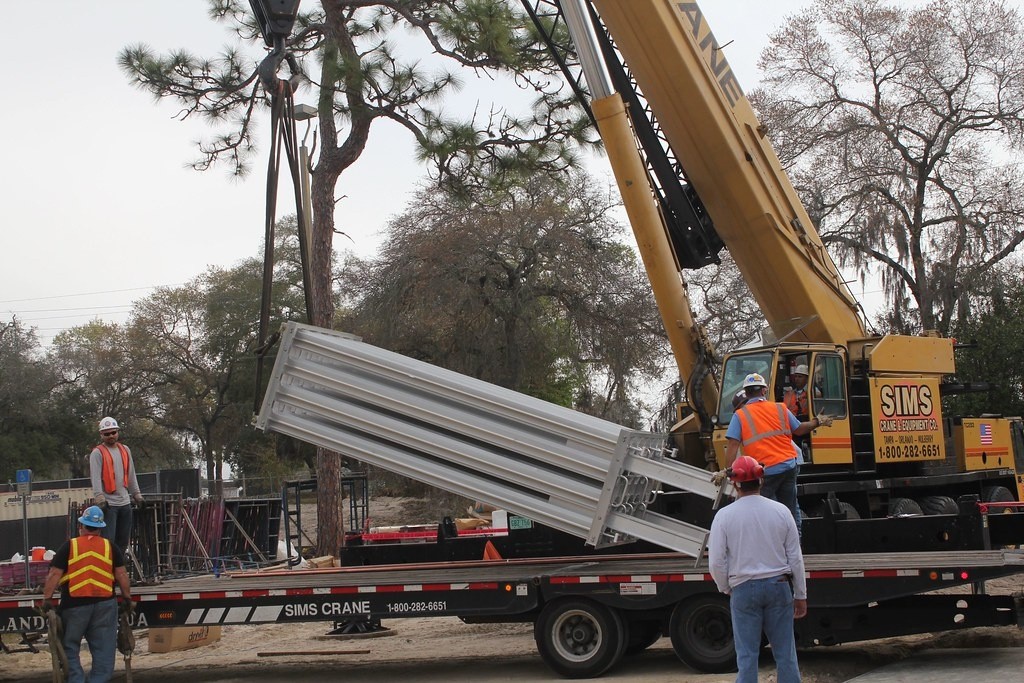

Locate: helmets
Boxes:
[726,456,765,482]
[742,373,768,389]
[732,389,749,414]
[98,416,120,432]
[78,506,107,527]
[794,365,809,376]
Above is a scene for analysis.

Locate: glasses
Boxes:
[102,431,118,436]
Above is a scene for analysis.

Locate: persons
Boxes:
[89,417,147,559]
[709,456,807,683]
[732,389,805,544]
[784,365,821,451]
[42,506,131,683]
[710,373,833,517]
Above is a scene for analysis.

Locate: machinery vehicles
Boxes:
[520,1,1024,545]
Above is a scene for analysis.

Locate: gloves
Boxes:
[710,470,727,486]
[40,599,54,619]
[812,407,833,427]
[98,500,110,521]
[132,493,147,513]
[120,598,133,614]
